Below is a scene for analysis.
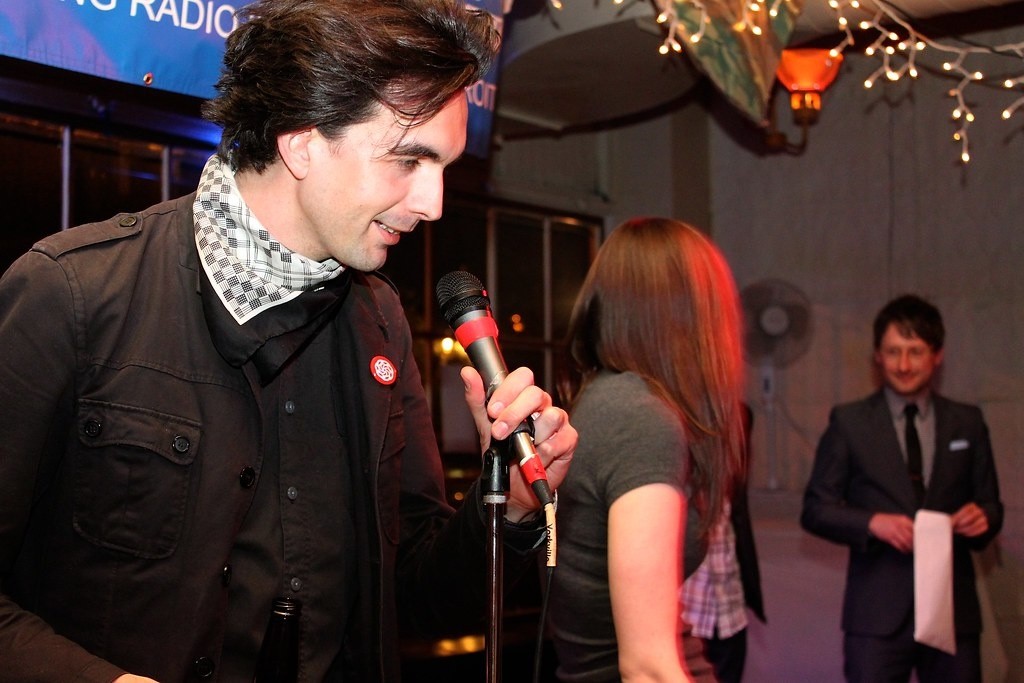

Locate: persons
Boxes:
[680,400,770,683]
[513,216,745,683]
[0,0,579,682]
[799,295,1005,683]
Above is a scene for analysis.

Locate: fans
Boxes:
[737,280,819,496]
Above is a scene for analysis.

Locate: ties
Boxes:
[904,403,923,498]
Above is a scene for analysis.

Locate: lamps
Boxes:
[763,48,846,154]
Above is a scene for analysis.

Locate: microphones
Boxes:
[437,270,553,506]
